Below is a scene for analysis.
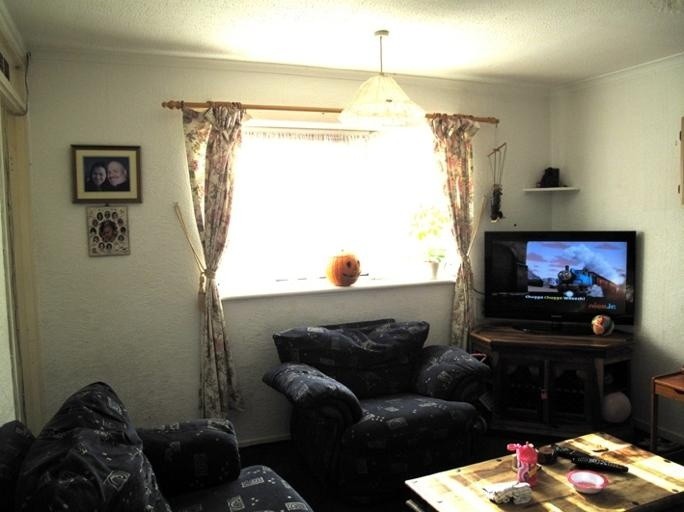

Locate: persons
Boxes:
[101,224,116,243]
[85,161,129,191]
[83,162,108,192]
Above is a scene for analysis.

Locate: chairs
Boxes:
[262,318,492,502]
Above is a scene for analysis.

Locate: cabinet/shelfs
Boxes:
[467,323,635,439]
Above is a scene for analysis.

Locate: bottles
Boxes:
[507,442,538,488]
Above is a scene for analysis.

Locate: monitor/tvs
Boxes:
[483,230,636,336]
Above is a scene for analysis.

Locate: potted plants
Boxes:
[413,206,447,280]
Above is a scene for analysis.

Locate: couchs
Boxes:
[0,414,314,512]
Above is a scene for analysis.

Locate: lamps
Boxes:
[337,30,426,129]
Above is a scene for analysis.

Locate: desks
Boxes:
[650,368,684,453]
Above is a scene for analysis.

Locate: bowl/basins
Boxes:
[568,470,608,495]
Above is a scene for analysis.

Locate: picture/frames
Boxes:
[70,144,142,204]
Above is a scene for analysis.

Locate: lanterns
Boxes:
[324,249,361,286]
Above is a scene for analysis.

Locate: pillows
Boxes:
[272,321,430,395]
[11,381,171,512]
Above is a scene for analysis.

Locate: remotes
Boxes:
[555,446,593,459]
[570,457,629,472]
